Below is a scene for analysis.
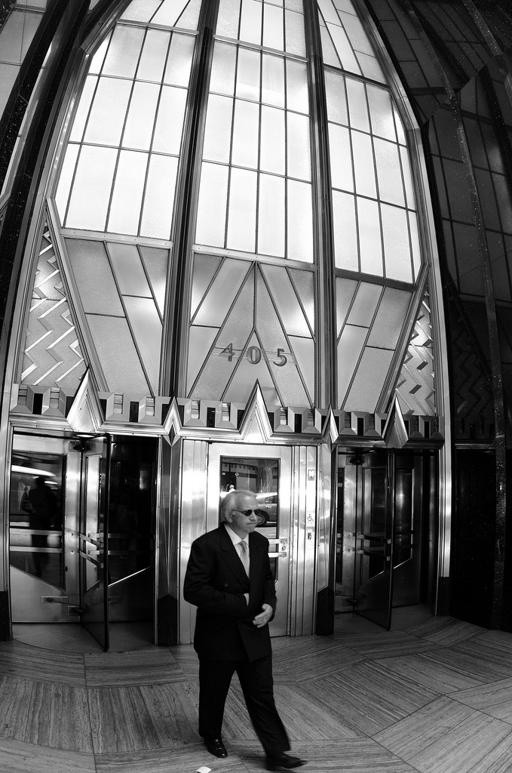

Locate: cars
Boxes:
[255,493,277,526]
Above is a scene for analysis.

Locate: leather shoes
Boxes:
[203,736,228,758]
[265,753,308,770]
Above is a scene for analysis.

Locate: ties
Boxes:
[238,541,251,578]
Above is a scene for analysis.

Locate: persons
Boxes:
[20,475,57,575]
[182,488,312,772]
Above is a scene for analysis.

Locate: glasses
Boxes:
[232,508,260,516]
